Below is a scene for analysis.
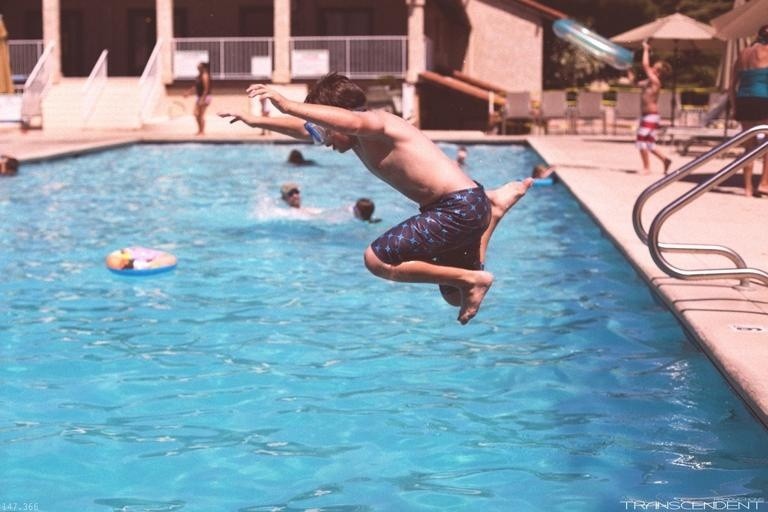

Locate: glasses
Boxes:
[305,121,327,147]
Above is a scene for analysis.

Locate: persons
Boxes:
[216,72,534,324]
[183,62,212,136]
[353,198,381,224]
[5,159,19,174]
[260,95,271,135]
[288,150,314,166]
[635,41,671,176]
[727,24,768,197]
[456,147,468,165]
[280,183,302,207]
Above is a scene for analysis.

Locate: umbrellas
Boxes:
[608,11,716,126]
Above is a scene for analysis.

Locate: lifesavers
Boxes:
[518,177,553,186]
[106,246,177,277]
[553,18,634,67]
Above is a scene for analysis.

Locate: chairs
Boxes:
[502,89,739,145]
